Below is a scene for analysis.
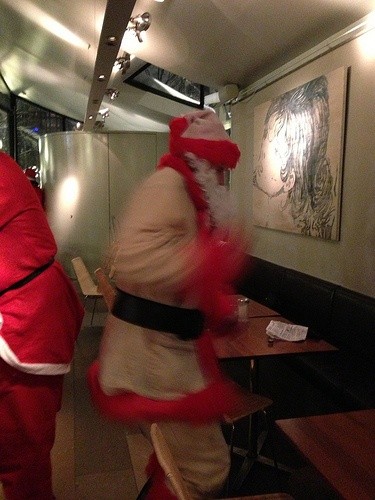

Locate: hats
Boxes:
[24,165,39,181]
[167,110,241,170]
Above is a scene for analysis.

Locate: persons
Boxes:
[0,141,85,500]
[24,168,43,205]
[87,109,248,500]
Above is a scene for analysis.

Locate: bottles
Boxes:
[236,298,249,322]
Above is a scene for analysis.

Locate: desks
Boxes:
[201,294,375,500]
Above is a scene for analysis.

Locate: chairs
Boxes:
[71,255,272,500]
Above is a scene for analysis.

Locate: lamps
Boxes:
[104,87,119,100]
[116,52,131,75]
[124,12,152,42]
